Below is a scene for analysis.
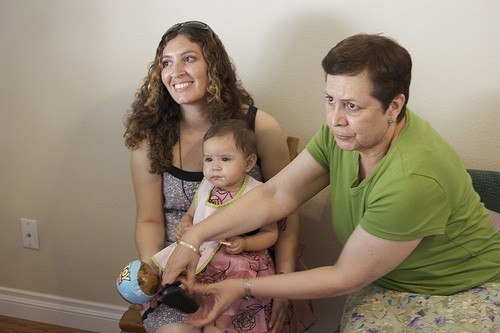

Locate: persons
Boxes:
[123,21,300,333]
[160,35,500,333]
[150,119,279,333]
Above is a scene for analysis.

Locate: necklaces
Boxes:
[179,122,212,203]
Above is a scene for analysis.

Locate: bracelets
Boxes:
[241,278,255,301]
[276,272,285,274]
[177,240,199,255]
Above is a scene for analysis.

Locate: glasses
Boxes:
[162,20,212,38]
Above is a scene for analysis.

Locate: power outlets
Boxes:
[20,219,40,251]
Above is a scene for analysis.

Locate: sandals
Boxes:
[158,281,200,314]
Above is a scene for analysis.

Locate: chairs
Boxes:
[119,137,299,333]
